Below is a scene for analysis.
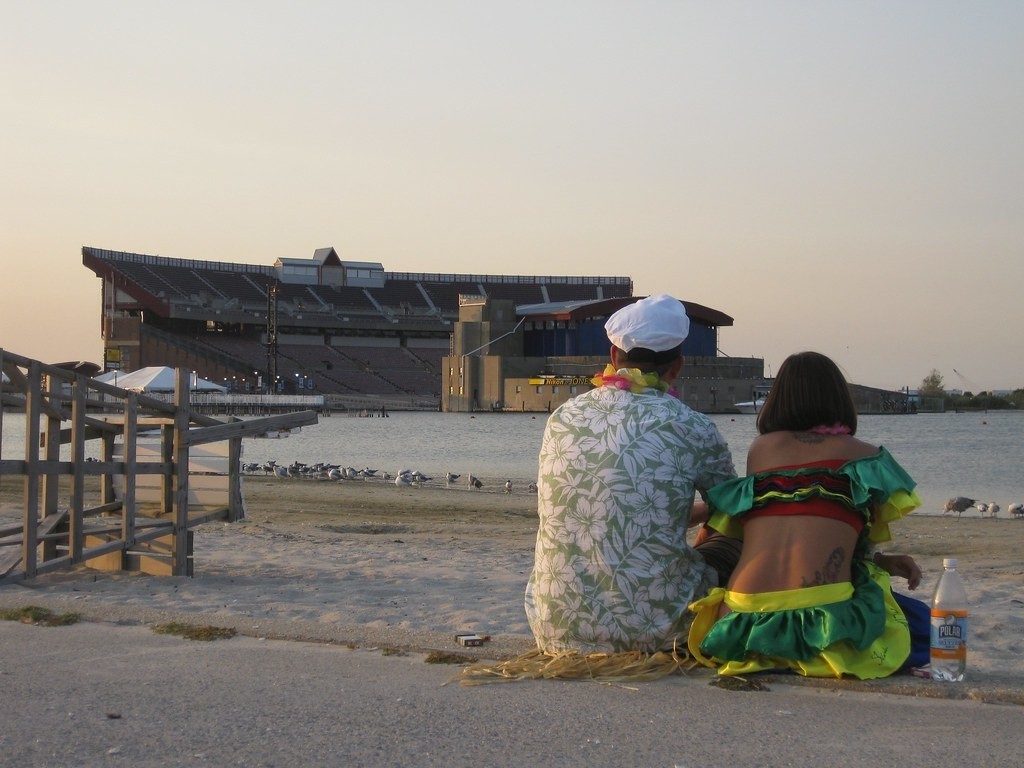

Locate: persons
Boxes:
[524,294,745,657]
[687,350,931,679]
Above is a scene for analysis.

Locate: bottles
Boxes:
[930,558,970,682]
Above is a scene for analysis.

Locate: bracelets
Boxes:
[870,547,882,562]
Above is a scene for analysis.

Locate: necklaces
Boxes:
[810,423,850,435]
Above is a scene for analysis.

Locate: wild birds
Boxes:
[85,456,102,463]
[940,496,1024,519]
[241,459,379,482]
[468,472,484,491]
[394,469,433,488]
[446,471,461,484]
[382,471,394,483]
[505,480,513,492]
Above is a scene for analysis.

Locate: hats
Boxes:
[604,294,690,353]
